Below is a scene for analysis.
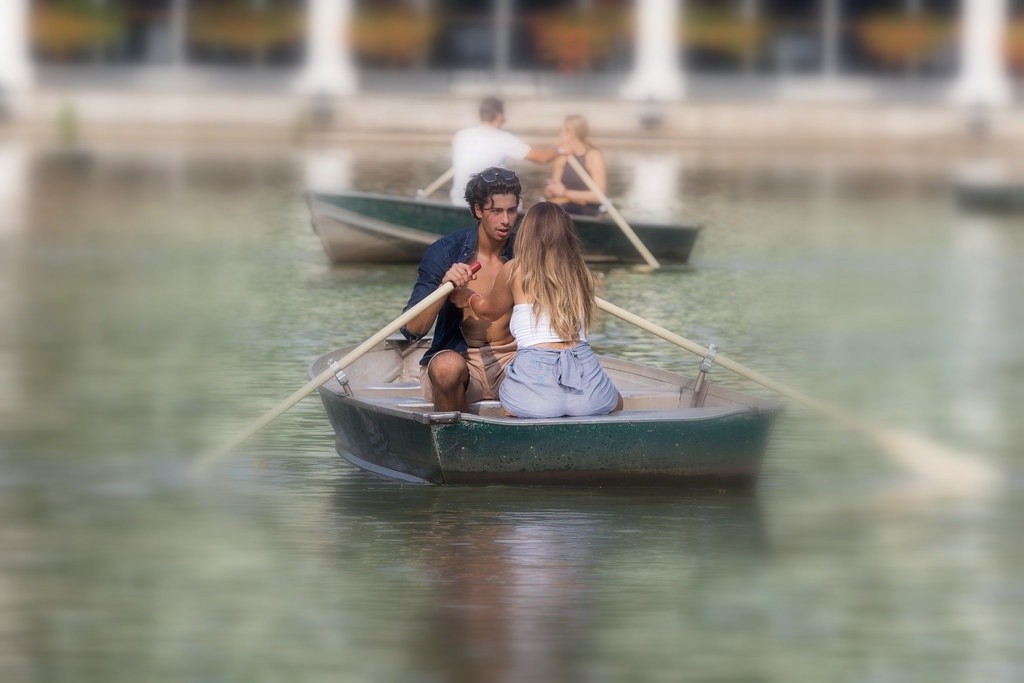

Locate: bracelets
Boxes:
[468,293,483,307]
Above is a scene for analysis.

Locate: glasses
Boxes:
[471,170,516,188]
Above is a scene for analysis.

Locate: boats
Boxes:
[949,178,1024,216]
[308,335,793,488]
[306,191,702,272]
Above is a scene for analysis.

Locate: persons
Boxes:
[401,167,530,413]
[449,97,574,206]
[449,201,624,419]
[544,113,607,217]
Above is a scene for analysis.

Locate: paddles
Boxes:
[568,154,660,268]
[594,297,983,491]
[186,262,482,471]
[422,166,455,198]
